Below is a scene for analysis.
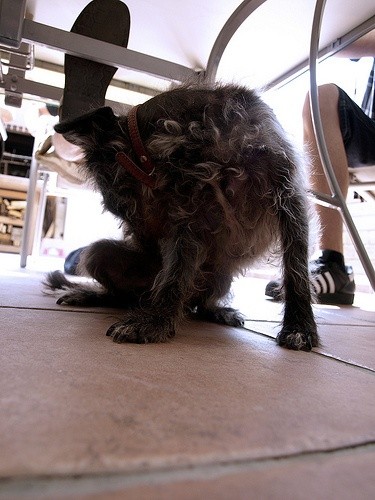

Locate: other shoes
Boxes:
[59,0,131,147]
[266,260,355,305]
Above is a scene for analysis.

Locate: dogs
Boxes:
[39,69,329,352]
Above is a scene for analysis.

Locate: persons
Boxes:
[34,0,131,185]
[266,29,375,306]
